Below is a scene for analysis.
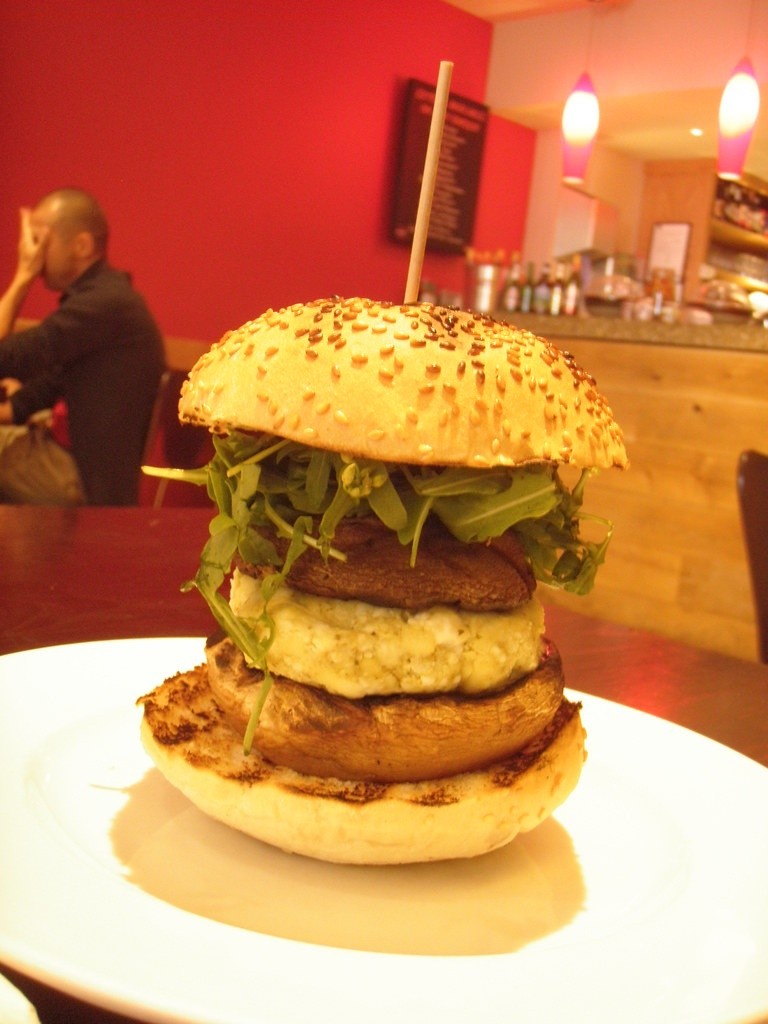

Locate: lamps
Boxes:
[561,0,600,184]
[716,0,760,182]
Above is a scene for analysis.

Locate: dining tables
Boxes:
[0,506,768,1024]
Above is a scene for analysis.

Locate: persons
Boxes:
[0,189,163,506]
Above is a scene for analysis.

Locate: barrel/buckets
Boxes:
[464,264,508,314]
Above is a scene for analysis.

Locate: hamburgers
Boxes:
[136,296,630,865]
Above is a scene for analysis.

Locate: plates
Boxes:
[0,638,768,1024]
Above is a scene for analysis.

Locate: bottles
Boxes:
[563,255,582,315]
[532,261,551,314]
[518,263,534,312]
[501,264,522,312]
[548,259,566,316]
